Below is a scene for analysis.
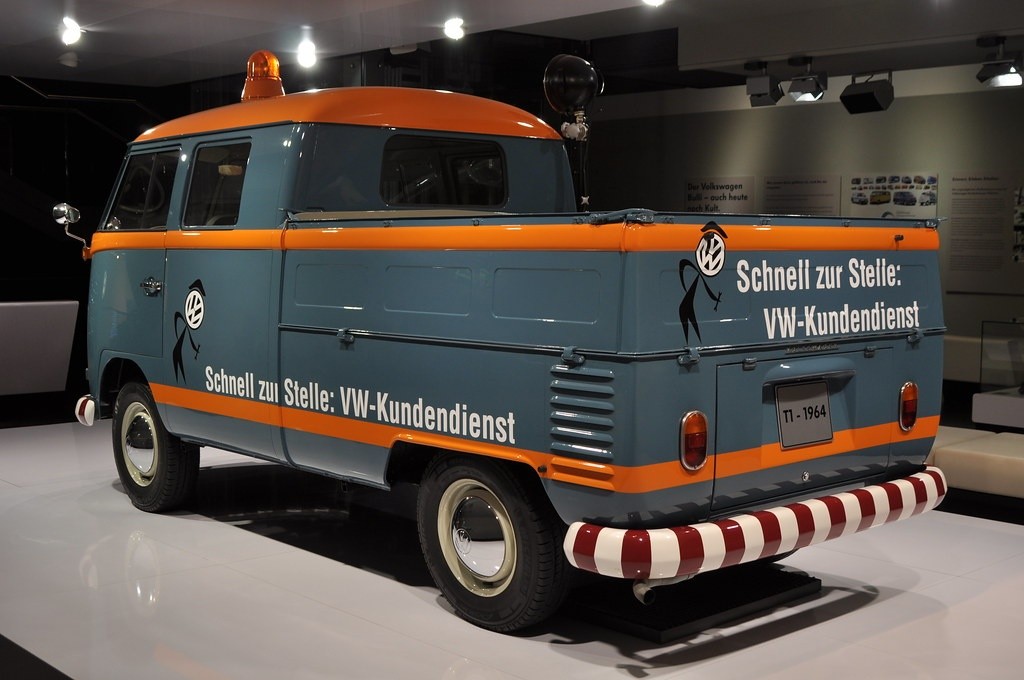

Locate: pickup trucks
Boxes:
[52,47,948,635]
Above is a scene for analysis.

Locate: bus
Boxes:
[870,190,891,204]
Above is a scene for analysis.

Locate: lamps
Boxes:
[976,36,1022,89]
[840,67,895,115]
[743,58,827,107]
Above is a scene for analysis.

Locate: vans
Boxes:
[894,191,916,205]
[852,192,868,204]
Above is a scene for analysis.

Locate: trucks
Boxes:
[851,175,936,185]
[918,191,936,206]
